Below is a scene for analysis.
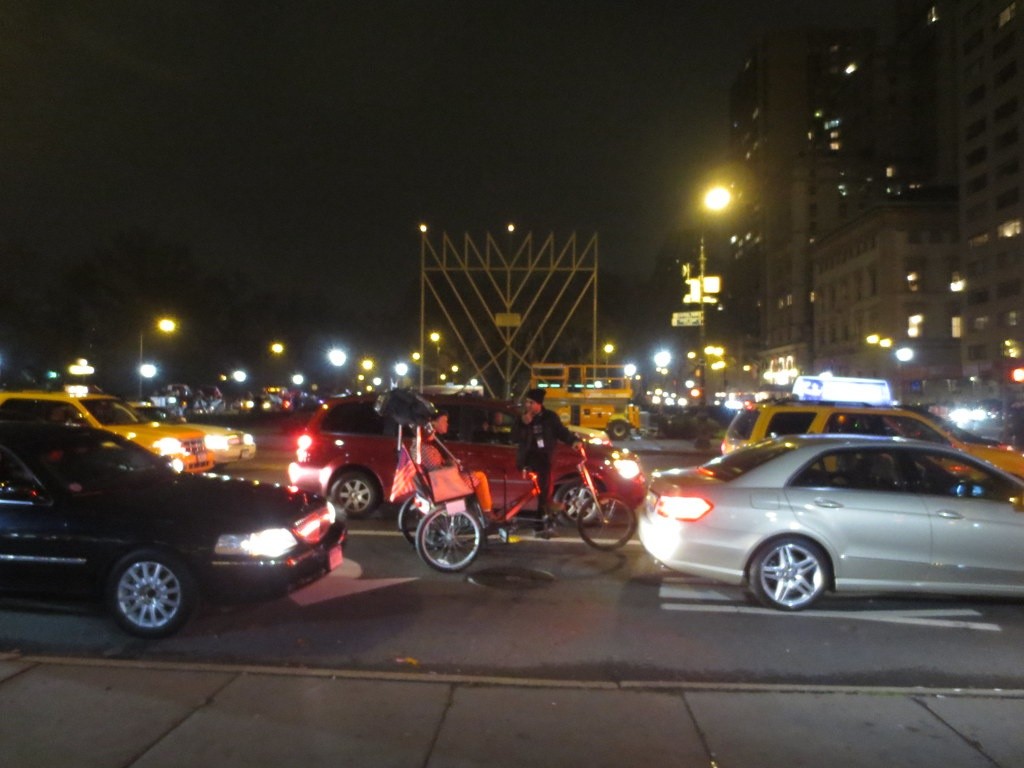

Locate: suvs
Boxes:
[0,387,215,474]
[722,370,1024,496]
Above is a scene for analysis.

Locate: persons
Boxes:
[239,387,272,415]
[834,454,871,489]
[291,391,301,408]
[168,384,222,417]
[510,388,584,536]
[878,453,899,491]
[471,411,509,441]
[409,410,501,520]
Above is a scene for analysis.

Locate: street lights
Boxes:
[691,185,727,447]
[135,320,176,398]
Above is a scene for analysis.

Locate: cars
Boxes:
[284,386,648,522]
[635,434,1024,615]
[0,418,347,640]
[126,398,257,467]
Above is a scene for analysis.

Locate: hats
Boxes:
[526,388,546,405]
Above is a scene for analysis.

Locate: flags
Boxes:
[390,447,416,503]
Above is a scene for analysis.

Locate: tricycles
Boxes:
[371,383,642,573]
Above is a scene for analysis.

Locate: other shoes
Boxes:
[533,529,559,539]
[484,509,504,525]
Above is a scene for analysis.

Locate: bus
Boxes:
[529,366,640,441]
